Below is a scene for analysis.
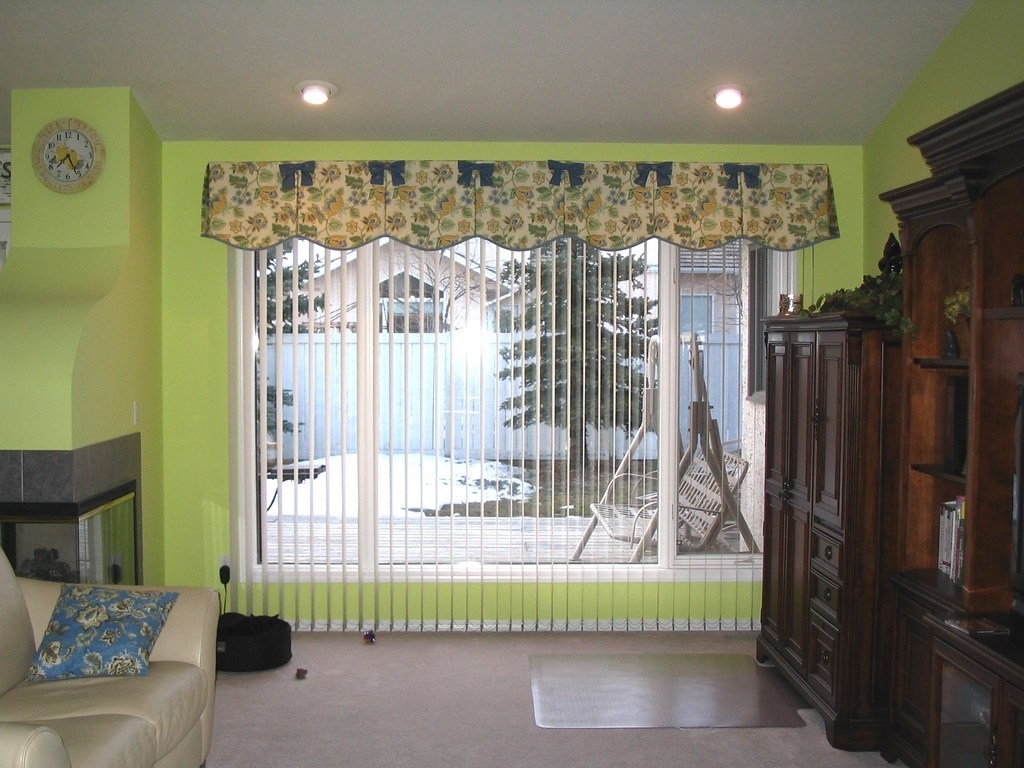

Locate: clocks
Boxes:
[31,117,107,194]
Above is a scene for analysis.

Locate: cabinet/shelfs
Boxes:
[761,311,885,752]
[879,81,1024,768]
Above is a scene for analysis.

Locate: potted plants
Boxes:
[943,289,971,360]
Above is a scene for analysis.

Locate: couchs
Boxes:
[0,548,221,768]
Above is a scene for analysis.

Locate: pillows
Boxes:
[24,584,180,681]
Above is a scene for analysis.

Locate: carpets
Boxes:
[527,651,814,730]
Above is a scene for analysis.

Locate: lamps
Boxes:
[706,84,751,110]
[293,80,339,105]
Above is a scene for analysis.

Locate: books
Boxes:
[938,495,965,589]
